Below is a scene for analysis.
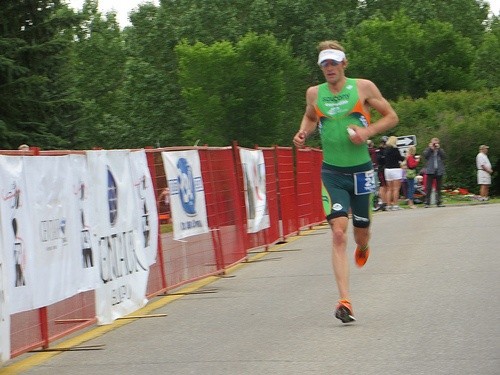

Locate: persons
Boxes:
[157,187,171,224]
[366,136,391,211]
[406,146,420,209]
[293,40,399,323]
[422,138,445,208]
[476,145,493,201]
[383,136,406,211]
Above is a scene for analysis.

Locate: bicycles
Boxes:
[370,180,385,212]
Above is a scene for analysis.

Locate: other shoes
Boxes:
[425,205,429,208]
[481,196,488,201]
[373,202,386,211]
[386,205,392,211]
[438,204,445,207]
[392,206,403,211]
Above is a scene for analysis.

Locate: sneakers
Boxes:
[354,243,370,267]
[335,300,356,324]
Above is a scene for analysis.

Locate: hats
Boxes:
[478,145,490,149]
[317,48,347,63]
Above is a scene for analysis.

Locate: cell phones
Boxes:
[347,128,356,135]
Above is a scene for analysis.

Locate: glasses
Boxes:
[319,59,342,65]
